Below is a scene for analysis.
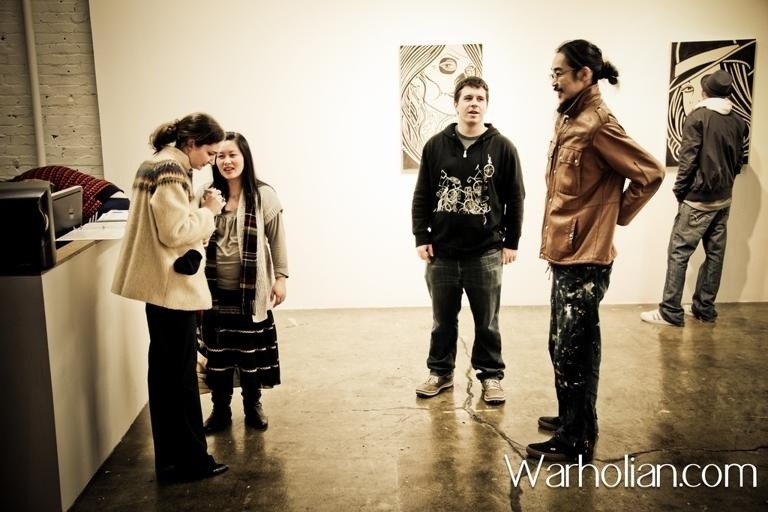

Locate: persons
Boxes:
[666,39,758,165]
[526,39,666,461]
[110,112,230,495]
[195,131,290,432]
[639,70,745,325]
[411,75,526,406]
[400,43,483,170]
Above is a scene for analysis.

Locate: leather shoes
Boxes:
[204,401,268,479]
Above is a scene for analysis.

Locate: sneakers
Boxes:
[640,305,696,326]
[526,416,593,462]
[416,370,454,397]
[481,377,506,403]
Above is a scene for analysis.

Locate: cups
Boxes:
[202,187,221,215]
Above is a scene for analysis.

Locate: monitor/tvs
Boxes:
[51,185,82,250]
[0,180,56,276]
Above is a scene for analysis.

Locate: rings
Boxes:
[221,197,227,202]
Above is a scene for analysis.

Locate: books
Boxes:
[97,209,128,221]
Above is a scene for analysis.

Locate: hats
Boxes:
[669,40,756,94]
[700,70,733,97]
